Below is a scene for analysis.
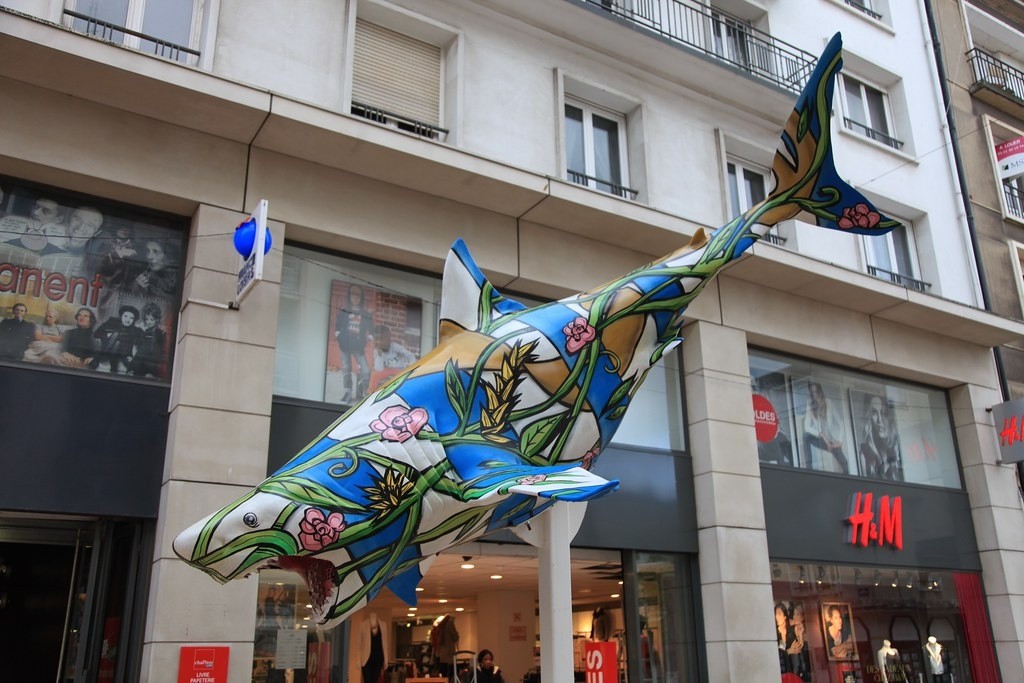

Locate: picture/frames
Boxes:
[819,602,861,662]
[251,581,298,660]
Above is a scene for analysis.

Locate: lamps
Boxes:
[797,565,937,591]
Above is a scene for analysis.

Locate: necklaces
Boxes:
[369,616,378,629]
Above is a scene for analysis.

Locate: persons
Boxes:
[918,636,954,683]
[751,374,903,483]
[430,613,459,677]
[774,600,856,683]
[878,639,908,683]
[589,607,611,642]
[335,284,416,404]
[357,612,388,683]
[471,649,505,683]
[1,198,179,378]
